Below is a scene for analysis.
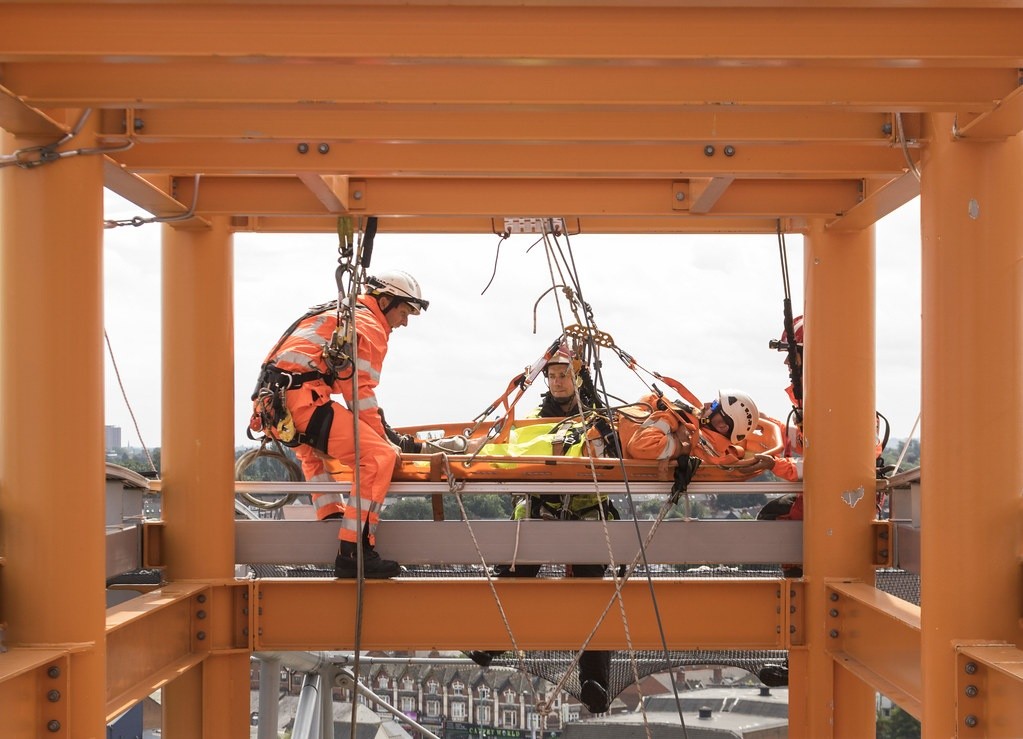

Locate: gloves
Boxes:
[673,399,692,421]
[656,398,668,410]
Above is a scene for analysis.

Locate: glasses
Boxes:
[710,400,726,416]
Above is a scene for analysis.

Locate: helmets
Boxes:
[719,390,759,445]
[543,352,569,377]
[365,269,429,315]
[770,314,804,351]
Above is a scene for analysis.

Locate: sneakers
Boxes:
[335,547,400,583]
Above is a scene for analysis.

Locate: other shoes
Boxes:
[759,659,788,687]
[460,650,495,667]
[581,680,609,713]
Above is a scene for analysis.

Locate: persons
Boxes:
[252,269,429,579]
[739,314,885,687]
[461,347,614,712]
[377,388,760,470]
